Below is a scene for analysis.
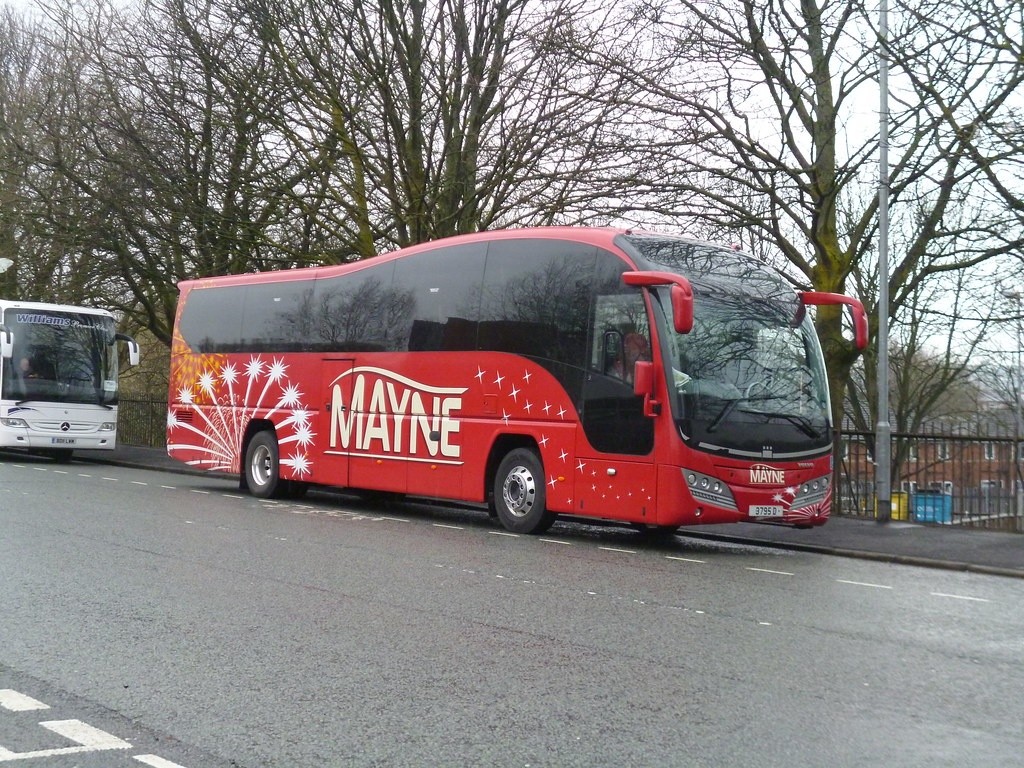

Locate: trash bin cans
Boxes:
[874,490,909,521]
[912,488,952,525]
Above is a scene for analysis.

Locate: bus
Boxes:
[0,299,141,459]
[168,225,869,536]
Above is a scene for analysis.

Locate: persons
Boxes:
[16,358,44,379]
[610,333,649,386]
[422,321,468,350]
